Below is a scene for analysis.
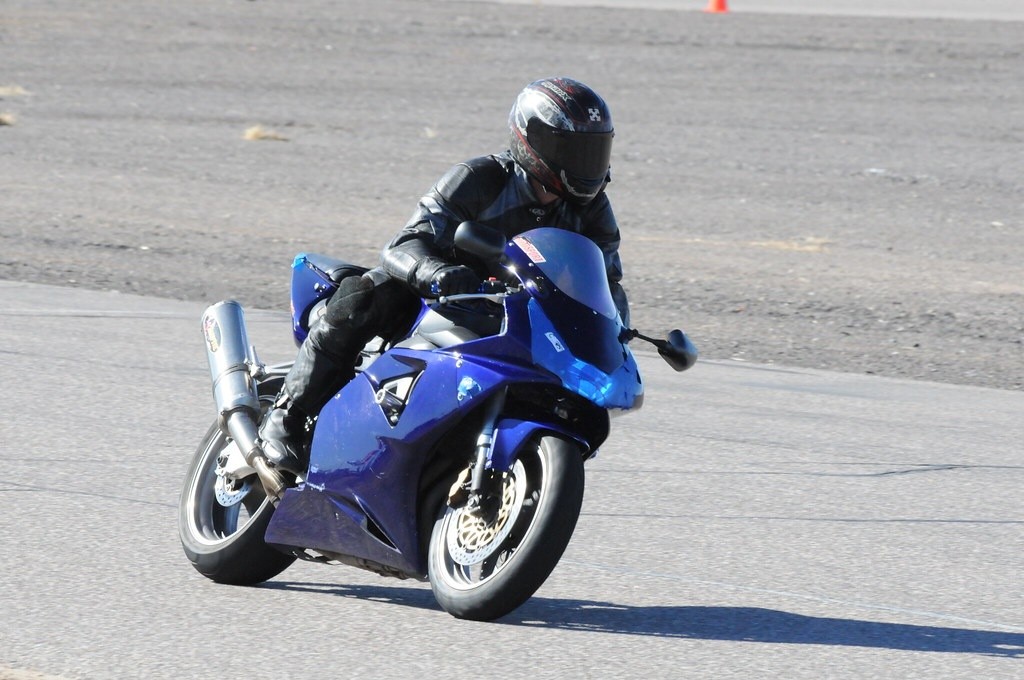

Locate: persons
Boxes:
[256,76,630,474]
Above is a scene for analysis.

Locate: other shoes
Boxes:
[257,405,302,467]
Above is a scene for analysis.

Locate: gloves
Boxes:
[432,264,483,296]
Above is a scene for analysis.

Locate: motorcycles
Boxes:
[177,220,697,622]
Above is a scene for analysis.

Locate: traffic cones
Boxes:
[704,1,730,13]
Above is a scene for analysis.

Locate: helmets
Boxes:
[509,76,615,206]
